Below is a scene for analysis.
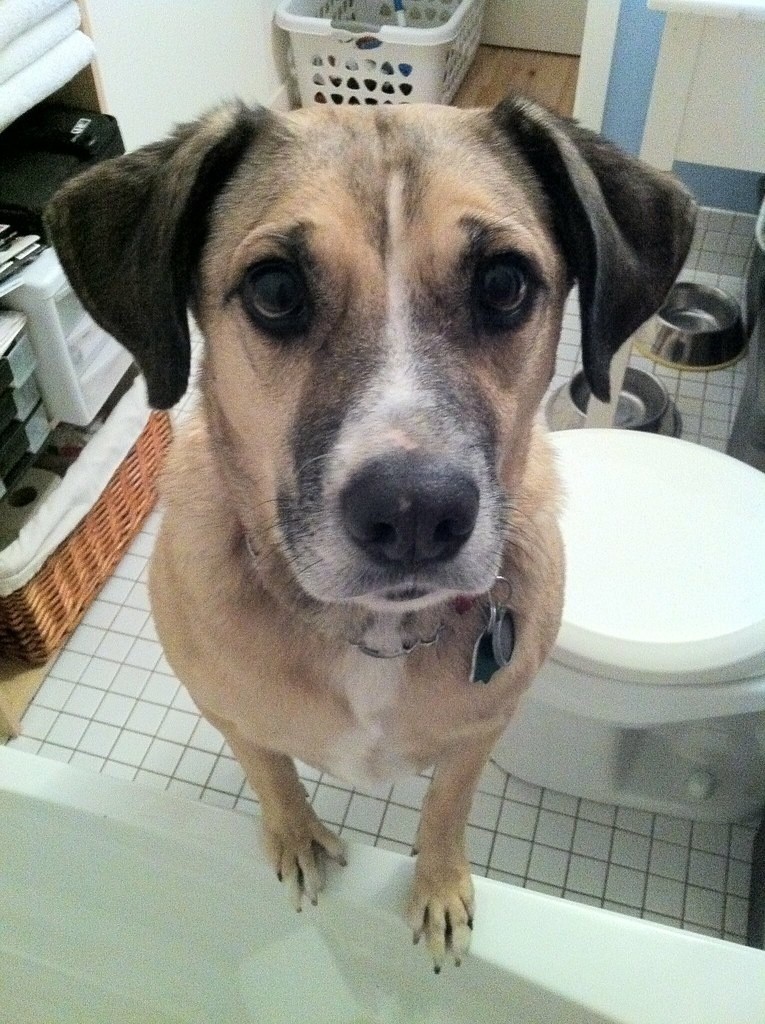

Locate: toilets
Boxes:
[488,424,765,826]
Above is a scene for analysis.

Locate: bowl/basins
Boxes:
[546,365,682,438]
[632,281,745,371]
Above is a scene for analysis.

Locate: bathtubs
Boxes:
[0,741,764,1024]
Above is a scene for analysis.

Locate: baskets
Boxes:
[272,1,484,110]
[0,369,176,668]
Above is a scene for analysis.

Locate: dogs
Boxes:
[42,90,699,974]
